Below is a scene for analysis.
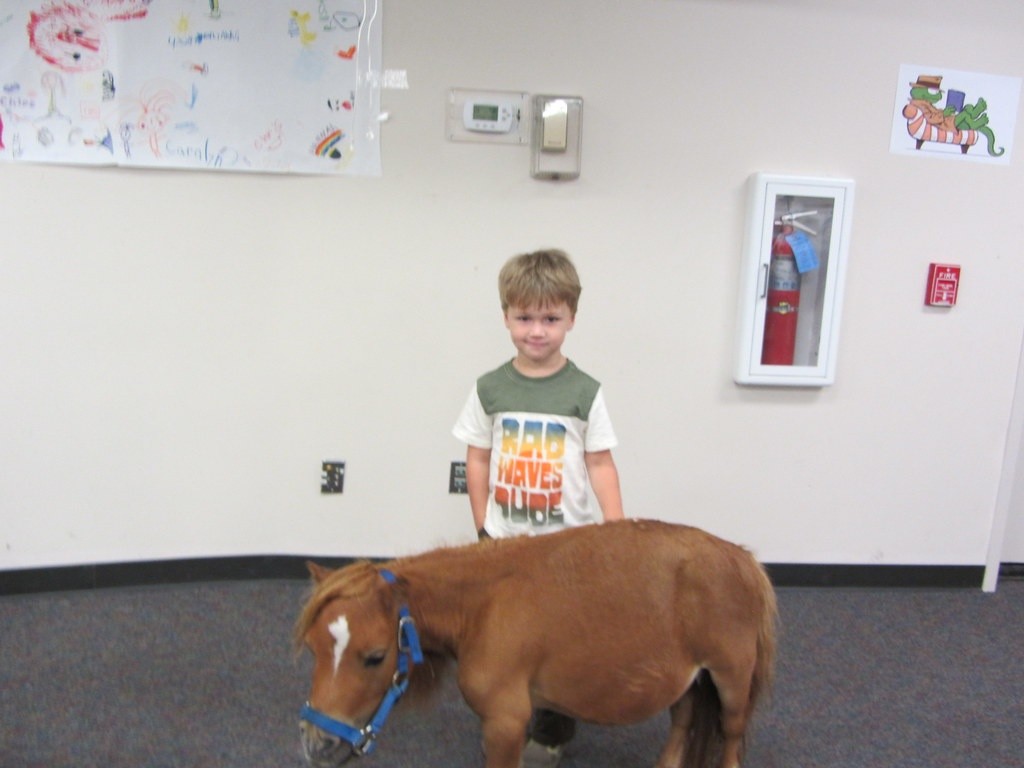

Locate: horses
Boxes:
[291,517,789,768]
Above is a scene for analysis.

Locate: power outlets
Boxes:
[321,461,344,492]
[450,462,469,494]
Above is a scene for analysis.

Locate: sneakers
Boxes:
[516,737,569,766]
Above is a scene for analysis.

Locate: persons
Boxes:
[452,248,626,766]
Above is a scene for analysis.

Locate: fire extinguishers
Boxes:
[760,209,818,366]
